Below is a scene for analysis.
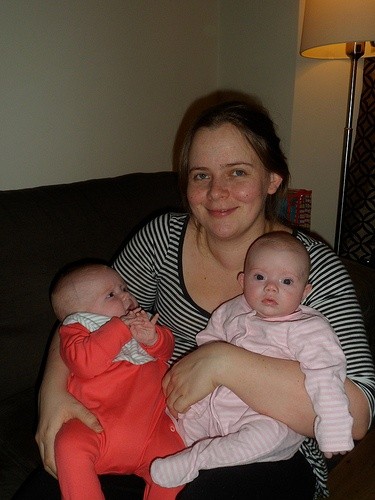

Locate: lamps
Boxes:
[297,1,375,268]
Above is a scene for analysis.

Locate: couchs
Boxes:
[0,169,204,500]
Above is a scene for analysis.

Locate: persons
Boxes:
[150,231,355,487]
[51,265,189,499]
[36,88,374,499]
